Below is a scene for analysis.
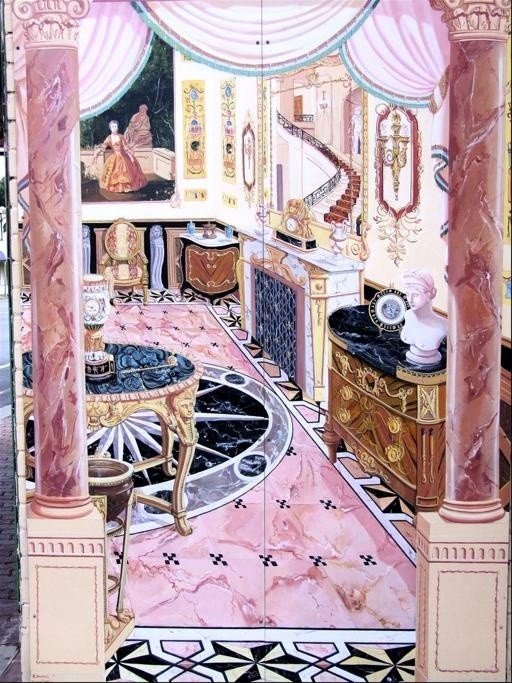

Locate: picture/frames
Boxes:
[79,28,181,207]
[254,51,372,263]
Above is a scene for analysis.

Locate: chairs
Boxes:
[97,214,151,305]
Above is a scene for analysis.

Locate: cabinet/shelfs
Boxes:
[315,302,450,516]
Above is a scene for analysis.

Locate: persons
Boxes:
[87,120,147,192]
[123,103,154,147]
[398,268,449,364]
[152,224,163,237]
[82,224,90,238]
[176,397,196,419]
[351,106,363,154]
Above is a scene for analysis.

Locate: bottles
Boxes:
[187,219,196,235]
[225,224,234,241]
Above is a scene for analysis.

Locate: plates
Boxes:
[367,287,409,333]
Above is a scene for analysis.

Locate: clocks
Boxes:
[82,270,111,349]
[281,200,314,239]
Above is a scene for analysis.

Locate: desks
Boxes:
[174,231,241,305]
[19,337,204,541]
